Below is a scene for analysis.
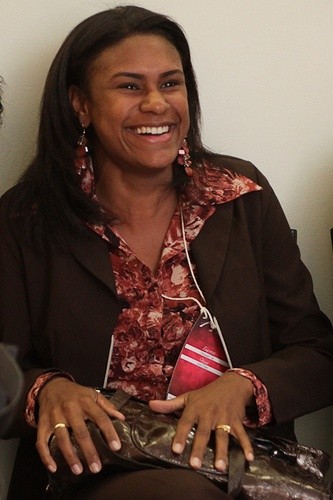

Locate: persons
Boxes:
[0,5,333,500]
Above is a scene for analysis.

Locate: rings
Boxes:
[53,423,69,432]
[215,424,231,433]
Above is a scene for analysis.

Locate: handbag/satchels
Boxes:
[44,385,333,500]
